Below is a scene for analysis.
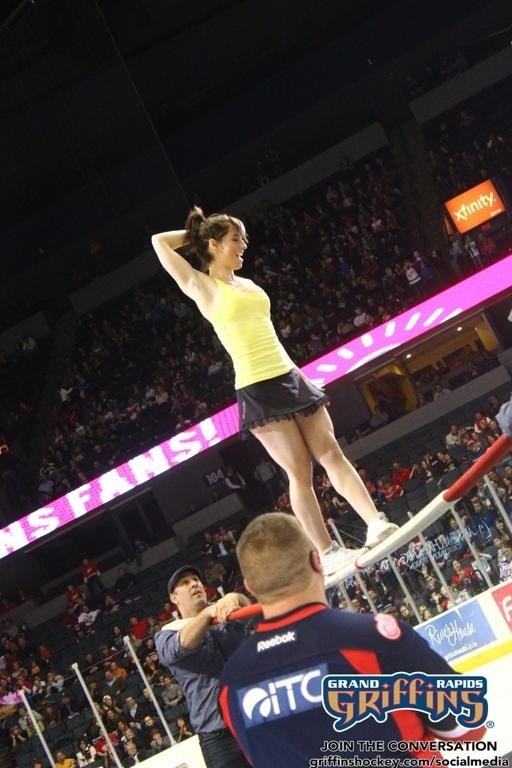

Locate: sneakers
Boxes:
[365,512,400,547]
[320,539,368,576]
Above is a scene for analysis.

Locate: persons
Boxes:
[242,87,511,369]
[216,512,487,767]
[0,554,195,768]
[276,382,512,624]
[0,284,234,530]
[154,565,250,766]
[0,410,512,767]
[150,205,399,576]
[197,525,236,602]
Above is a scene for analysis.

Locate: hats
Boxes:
[168,565,202,595]
